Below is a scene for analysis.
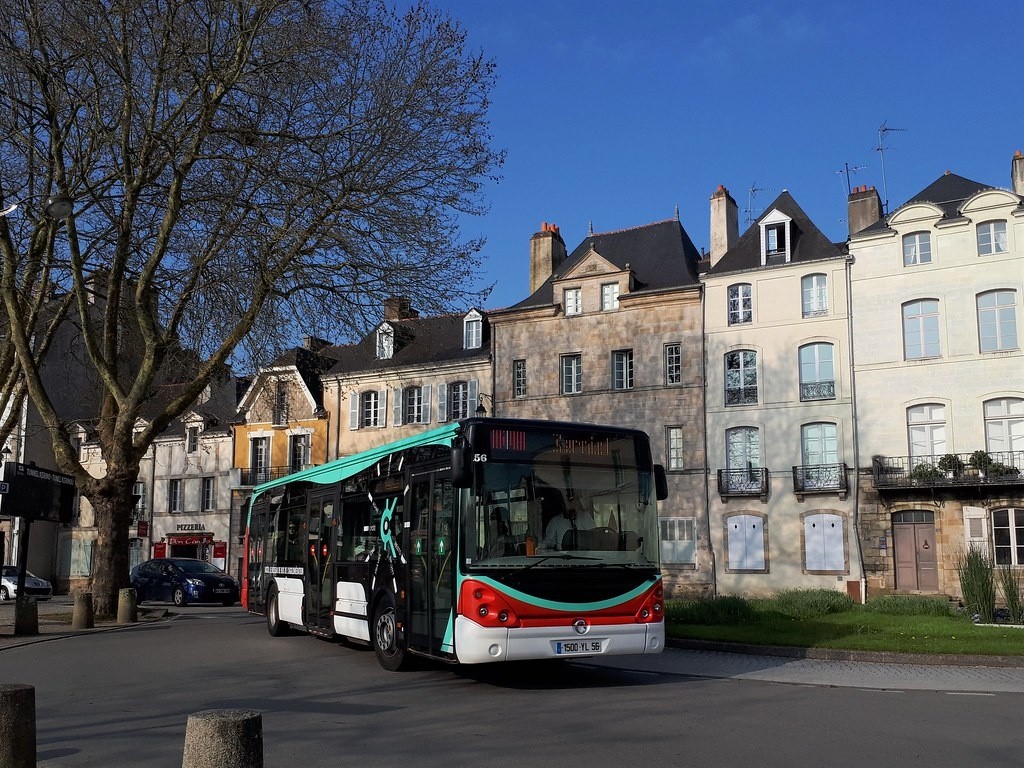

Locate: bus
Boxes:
[239,416,667,673]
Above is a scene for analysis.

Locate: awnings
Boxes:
[165,532,214,545]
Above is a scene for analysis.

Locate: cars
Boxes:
[0,565,53,601]
[131,557,240,607]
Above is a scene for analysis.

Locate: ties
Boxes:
[571,518,577,530]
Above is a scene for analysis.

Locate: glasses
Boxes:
[289,527,295,530]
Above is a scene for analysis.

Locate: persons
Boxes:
[162,564,184,574]
[540,494,596,551]
[354,536,378,561]
[486,523,515,560]
[277,520,300,567]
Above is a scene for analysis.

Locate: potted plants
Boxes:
[905,450,1020,482]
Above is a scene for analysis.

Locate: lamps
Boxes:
[474,393,492,417]
